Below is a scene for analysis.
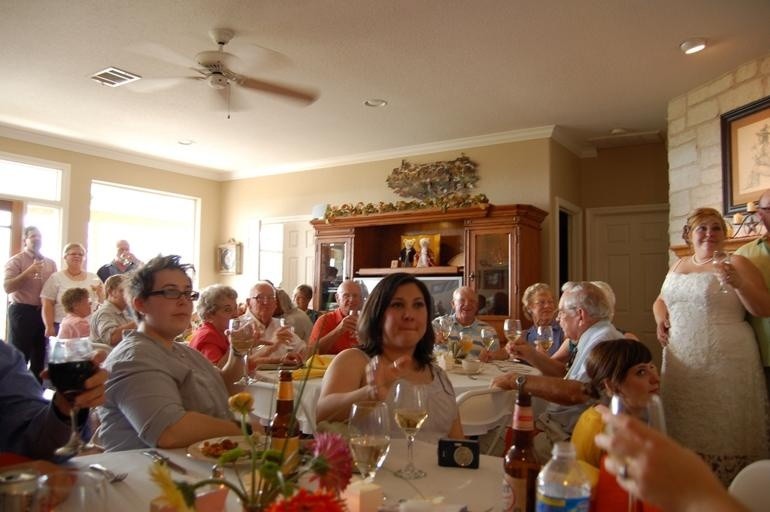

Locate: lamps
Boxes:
[682,38,709,57]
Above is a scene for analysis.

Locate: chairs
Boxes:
[454,384,520,459]
[727,458,769,512]
[646,392,667,435]
[237,377,319,435]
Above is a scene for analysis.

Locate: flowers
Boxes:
[147,391,354,512]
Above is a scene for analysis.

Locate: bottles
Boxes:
[209,463,224,489]
[535,442,591,512]
[269,369,300,483]
[500,390,541,512]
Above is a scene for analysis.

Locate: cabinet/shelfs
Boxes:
[310,204,548,349]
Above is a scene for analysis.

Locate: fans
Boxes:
[126,25,321,114]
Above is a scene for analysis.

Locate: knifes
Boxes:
[140,450,188,476]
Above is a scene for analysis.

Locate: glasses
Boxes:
[143,289,200,301]
[254,295,276,304]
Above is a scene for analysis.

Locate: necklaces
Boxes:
[692,253,714,266]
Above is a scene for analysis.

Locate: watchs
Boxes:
[515,373,527,393]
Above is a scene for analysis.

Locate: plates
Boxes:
[185,434,272,468]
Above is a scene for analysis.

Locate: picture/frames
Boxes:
[215,236,243,278]
[719,94,770,218]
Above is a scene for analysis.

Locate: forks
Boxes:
[88,463,129,484]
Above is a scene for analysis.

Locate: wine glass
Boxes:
[90,277,100,298]
[393,380,429,479]
[608,392,666,512]
[46,336,106,458]
[347,400,392,501]
[227,310,554,387]
[31,469,108,512]
[712,250,731,295]
[121,253,133,266]
[32,258,43,280]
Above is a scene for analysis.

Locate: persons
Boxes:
[571,338,661,512]
[732,187,770,382]
[39,240,144,355]
[4,226,57,386]
[1,340,108,464]
[653,207,770,488]
[430,286,500,360]
[594,411,770,512]
[315,273,464,445]
[476,277,640,367]
[185,279,364,368]
[487,281,627,465]
[98,253,274,454]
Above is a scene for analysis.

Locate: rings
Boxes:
[727,274,730,279]
[618,464,627,478]
